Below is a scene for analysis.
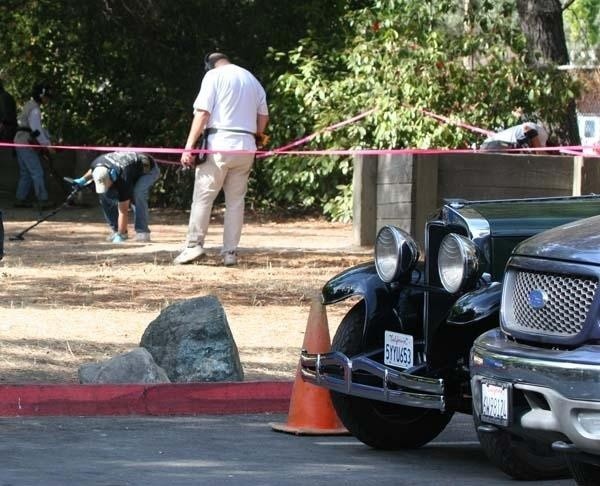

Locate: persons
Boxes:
[478,121,552,157]
[71,151,162,242]
[172,50,270,265]
[12,83,59,213]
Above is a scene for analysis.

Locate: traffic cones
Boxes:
[269,294,352,435]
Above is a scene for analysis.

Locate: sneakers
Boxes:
[223,251,237,264]
[172,243,206,263]
[105,231,127,242]
[135,232,150,240]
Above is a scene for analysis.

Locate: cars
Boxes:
[299,194,600,456]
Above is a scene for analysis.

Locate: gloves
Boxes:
[73,177,85,189]
[113,233,126,242]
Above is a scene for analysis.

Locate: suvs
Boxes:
[468,216,600,476]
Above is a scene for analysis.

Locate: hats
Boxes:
[92,166,110,193]
[204,48,228,61]
[38,85,51,98]
